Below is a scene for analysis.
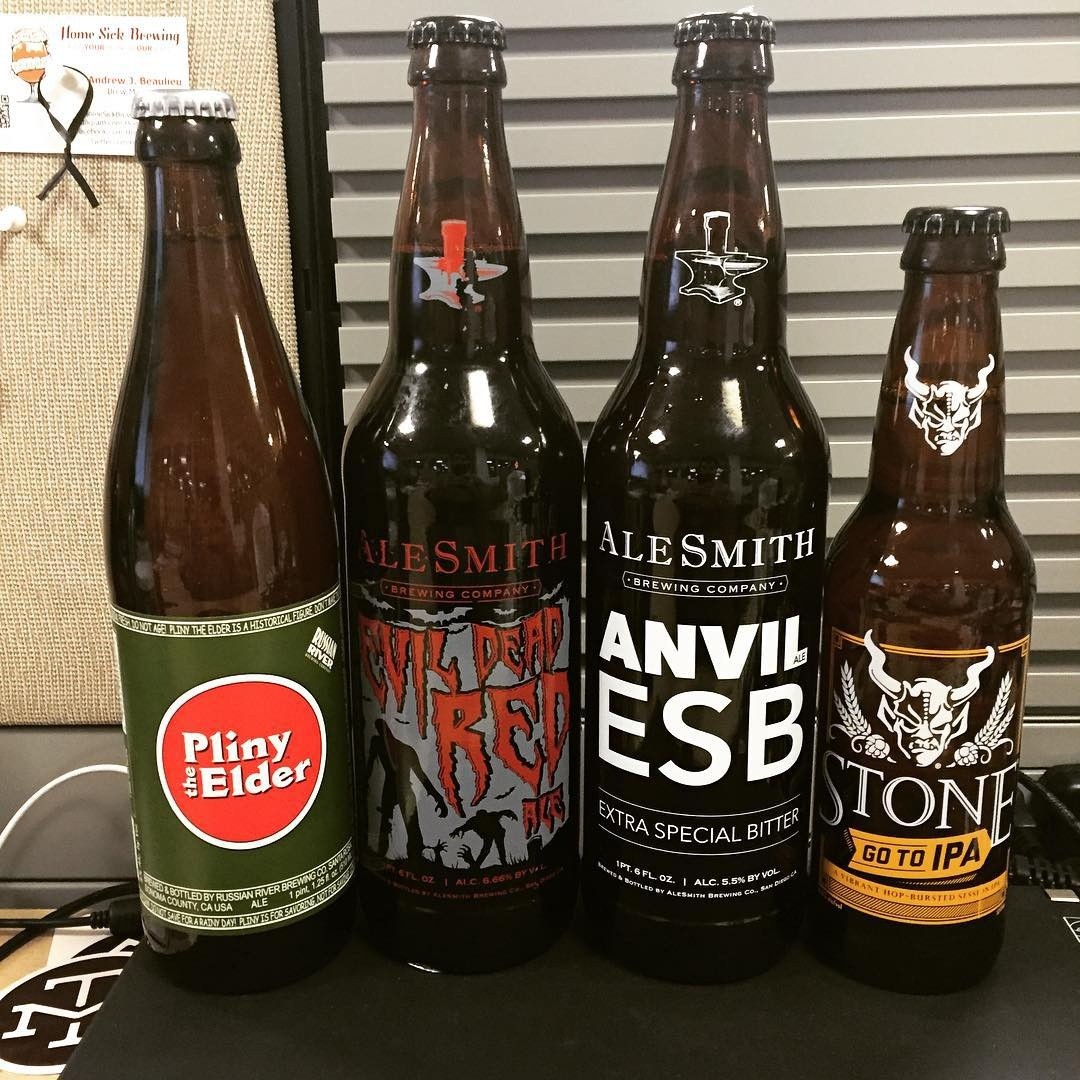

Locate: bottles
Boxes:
[582,15,834,982]
[97,88,336,997]
[336,18,583,976]
[804,203,1040,992]
